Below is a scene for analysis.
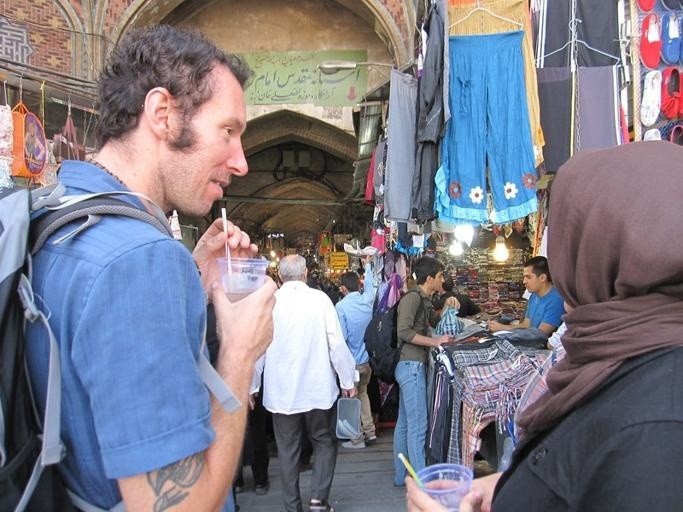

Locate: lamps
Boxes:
[317,59,395,75]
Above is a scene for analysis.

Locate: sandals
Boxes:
[309,499,335,512]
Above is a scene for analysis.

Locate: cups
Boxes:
[218,257,269,304]
[411,462,474,511]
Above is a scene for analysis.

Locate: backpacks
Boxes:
[0,182,174,512]
[363,290,423,385]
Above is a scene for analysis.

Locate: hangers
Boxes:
[535,19,622,71]
[448,0,523,35]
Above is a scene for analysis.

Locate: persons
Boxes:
[201,252,481,512]
[26,122,44,160]
[0,26,281,512]
[407,142,683,512]
[488,256,563,337]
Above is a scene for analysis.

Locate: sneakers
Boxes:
[235,486,243,493]
[256,480,269,494]
[365,432,377,443]
[341,437,366,449]
